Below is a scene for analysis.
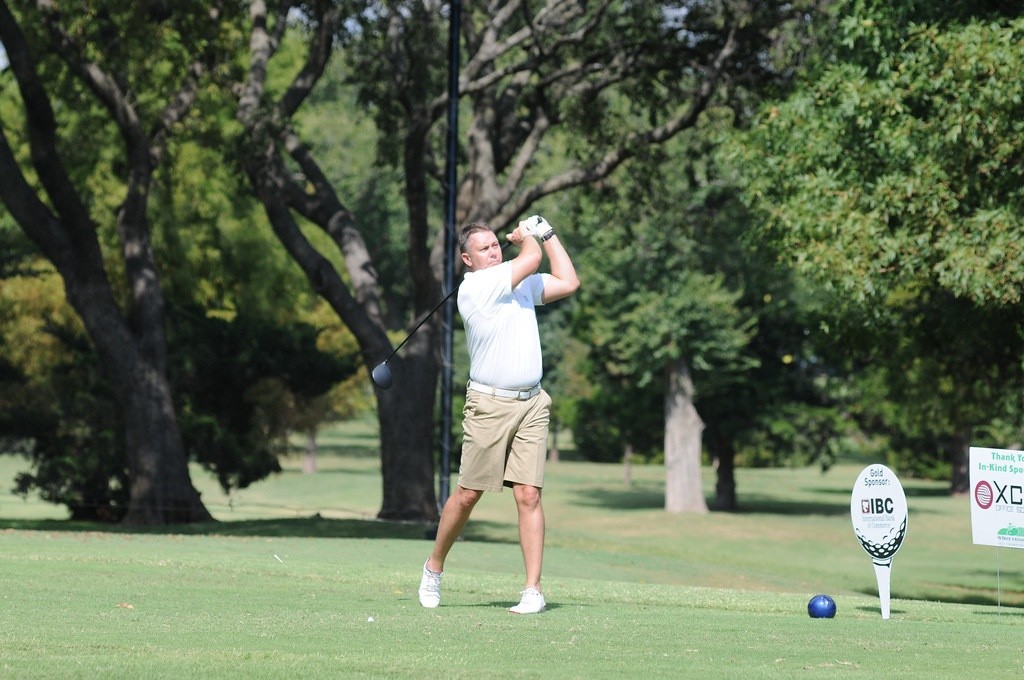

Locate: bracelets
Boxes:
[541,231,556,243]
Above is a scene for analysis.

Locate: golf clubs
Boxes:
[369,216,546,392]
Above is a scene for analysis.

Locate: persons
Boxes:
[417,214,582,616]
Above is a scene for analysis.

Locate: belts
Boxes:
[468,381,545,401]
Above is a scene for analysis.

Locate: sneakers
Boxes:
[418,557,443,608]
[509,587,547,615]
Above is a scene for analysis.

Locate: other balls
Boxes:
[808,594,837,619]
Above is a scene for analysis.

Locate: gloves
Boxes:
[517,215,552,239]
